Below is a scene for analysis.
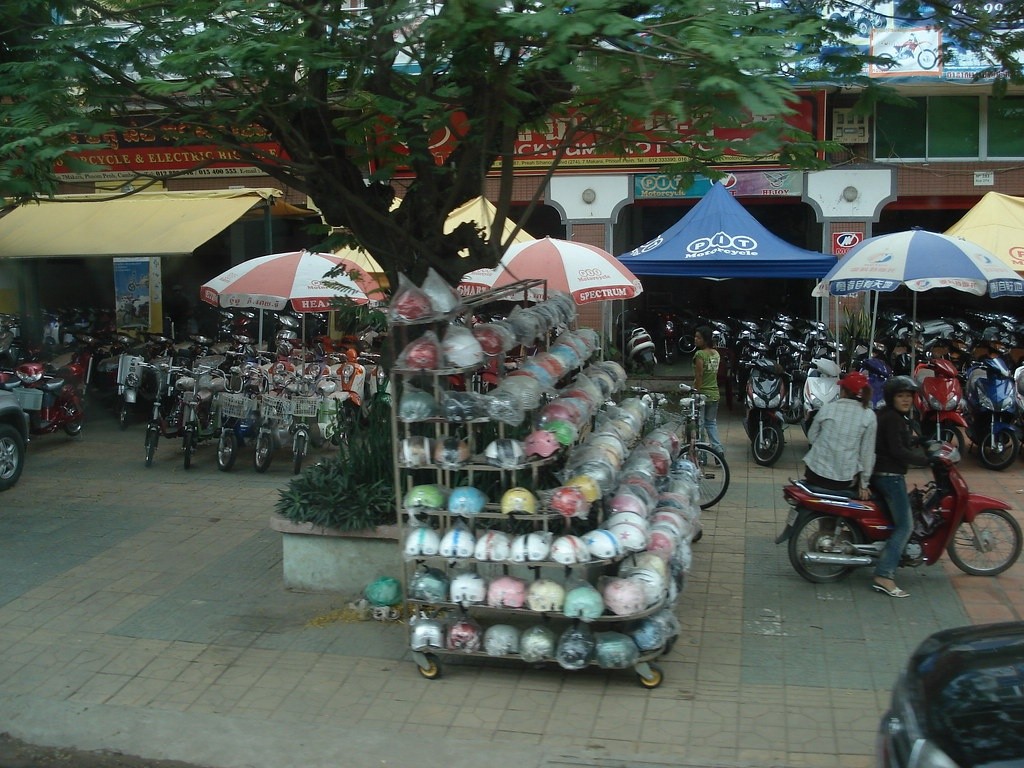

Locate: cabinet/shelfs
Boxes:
[388,280,703,688]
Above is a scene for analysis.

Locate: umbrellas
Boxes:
[943,191,1024,279]
[199,248,387,392]
[811,224,1024,378]
[455,233,644,351]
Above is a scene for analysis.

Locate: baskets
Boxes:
[260,393,292,420]
[13,387,43,410]
[658,409,685,433]
[222,393,253,419]
[293,396,319,417]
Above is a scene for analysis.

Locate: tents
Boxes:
[0,187,322,367]
[614,181,840,367]
[326,193,581,346]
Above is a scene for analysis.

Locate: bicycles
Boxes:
[674,382,732,510]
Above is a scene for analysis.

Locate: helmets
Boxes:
[366,280,700,671]
[881,375,918,402]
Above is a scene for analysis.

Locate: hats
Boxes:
[837,372,869,394]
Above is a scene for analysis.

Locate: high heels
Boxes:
[872,582,910,598]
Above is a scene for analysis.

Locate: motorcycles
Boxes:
[772,421,1024,585]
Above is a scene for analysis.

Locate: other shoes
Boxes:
[714,452,724,465]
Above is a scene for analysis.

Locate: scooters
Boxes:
[615,300,1024,473]
[0,304,393,494]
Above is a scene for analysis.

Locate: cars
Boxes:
[875,622,1024,768]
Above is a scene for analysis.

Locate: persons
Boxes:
[800,370,877,533]
[872,374,935,598]
[693,325,726,465]
